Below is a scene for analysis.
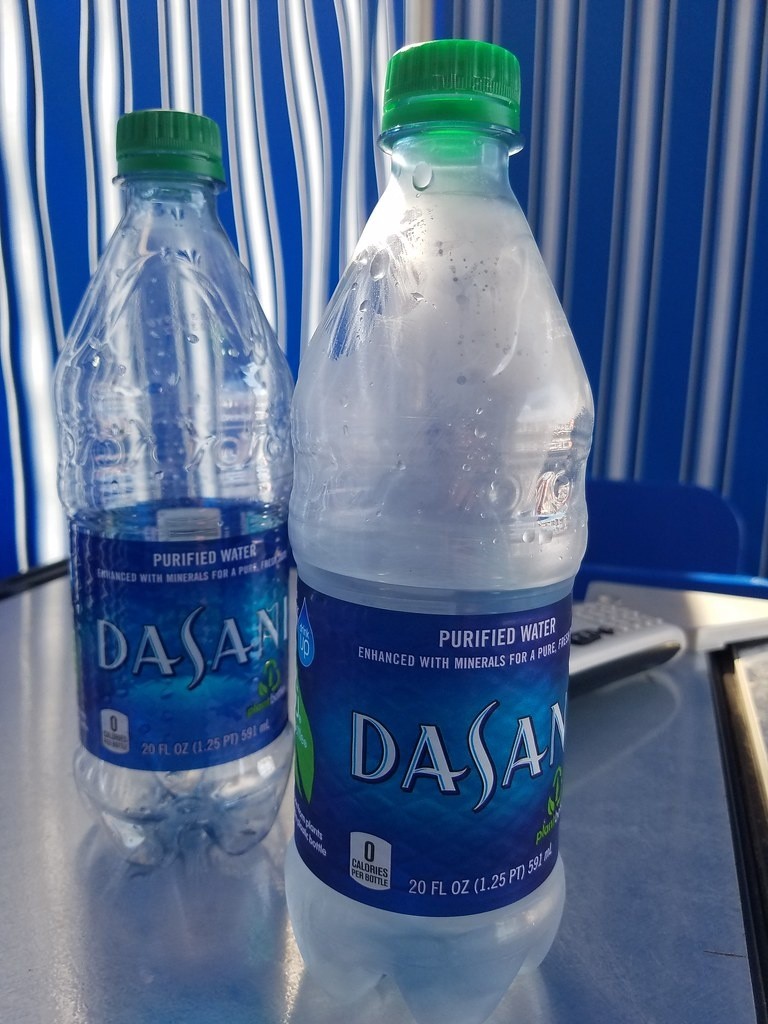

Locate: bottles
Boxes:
[293,40,595,1024]
[54,108,295,870]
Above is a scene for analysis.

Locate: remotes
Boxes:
[566,600,687,699]
